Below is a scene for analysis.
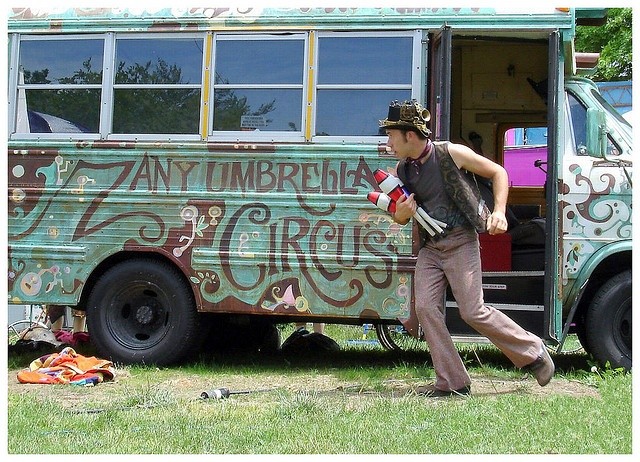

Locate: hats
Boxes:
[379,99,432,137]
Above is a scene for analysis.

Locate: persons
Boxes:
[377,102,555,397]
[48,305,86,333]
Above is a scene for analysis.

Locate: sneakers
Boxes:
[529,339,555,387]
[408,381,470,397]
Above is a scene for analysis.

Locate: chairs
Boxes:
[470,132,545,248]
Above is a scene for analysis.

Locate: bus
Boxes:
[5,4,631,371]
